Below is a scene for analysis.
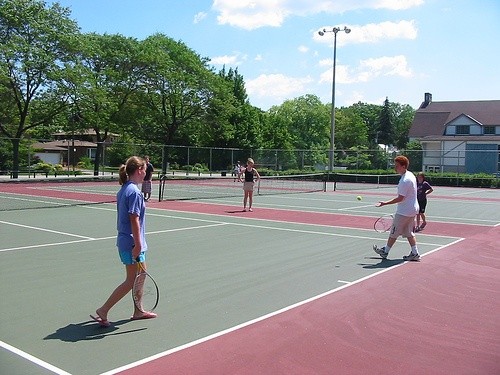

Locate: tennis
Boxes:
[357,196,362,201]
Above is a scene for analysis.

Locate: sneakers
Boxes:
[249,207,253,212]
[420,222,426,228]
[414,226,421,232]
[243,208,247,212]
[403,251,422,261]
[373,244,388,259]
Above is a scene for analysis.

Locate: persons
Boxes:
[416,172,434,233]
[233,160,244,183]
[141,156,154,200]
[238,158,261,212]
[90,156,157,327]
[372,155,422,261]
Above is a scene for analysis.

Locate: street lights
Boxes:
[318,26,351,171]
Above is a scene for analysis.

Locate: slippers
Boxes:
[130,312,158,319]
[90,314,111,326]
